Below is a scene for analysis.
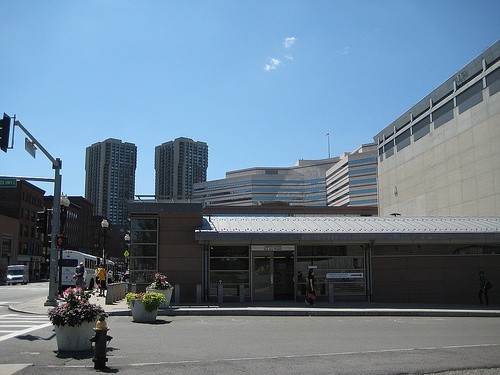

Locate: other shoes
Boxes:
[102,294,105,297]
[97,295,102,297]
[305,299,310,305]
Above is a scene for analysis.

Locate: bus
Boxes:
[53,250,115,290]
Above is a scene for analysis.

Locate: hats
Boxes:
[80,262,83,264]
[77,266,80,268]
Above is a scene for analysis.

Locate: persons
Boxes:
[108,269,113,284]
[479,271,489,305]
[304,269,315,307]
[114,272,121,282]
[94,264,106,297]
[73,262,87,292]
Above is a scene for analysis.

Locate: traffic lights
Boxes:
[36,206,48,234]
[0,112,14,154]
[57,236,69,247]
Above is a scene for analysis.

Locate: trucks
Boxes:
[6,264,30,285]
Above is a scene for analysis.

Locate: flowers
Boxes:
[47,286,112,327]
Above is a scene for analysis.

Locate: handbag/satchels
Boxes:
[310,294,316,300]
[485,281,492,291]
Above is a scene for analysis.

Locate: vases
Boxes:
[149,271,171,290]
[145,286,174,309]
[50,314,98,353]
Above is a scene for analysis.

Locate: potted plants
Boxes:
[124,290,166,322]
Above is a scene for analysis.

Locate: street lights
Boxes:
[124,233,131,271]
[101,218,110,270]
[59,192,70,298]
